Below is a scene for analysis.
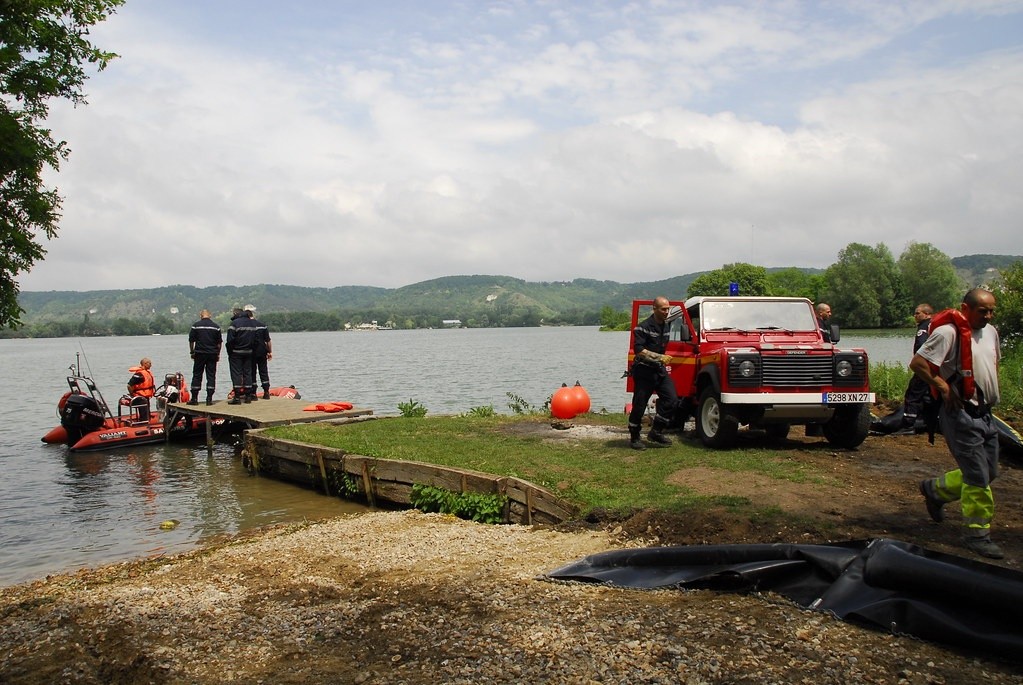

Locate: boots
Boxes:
[243,385,252,403]
[647,413,673,447]
[228,386,242,404]
[186,386,201,405]
[891,413,918,435]
[628,421,646,450]
[261,381,270,399]
[251,383,258,401]
[206,386,215,406]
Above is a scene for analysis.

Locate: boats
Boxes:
[40,350,303,453]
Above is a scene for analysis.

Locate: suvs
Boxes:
[626,282,877,449]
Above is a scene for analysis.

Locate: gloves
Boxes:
[638,349,673,365]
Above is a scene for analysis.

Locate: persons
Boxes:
[244,310,273,401]
[127,358,155,422]
[911,288,1004,558]
[627,297,677,450]
[225,308,256,405]
[892,304,934,435]
[154,373,189,423]
[187,310,223,404]
[816,303,832,343]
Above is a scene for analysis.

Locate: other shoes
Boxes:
[919,479,946,523]
[959,537,1005,559]
